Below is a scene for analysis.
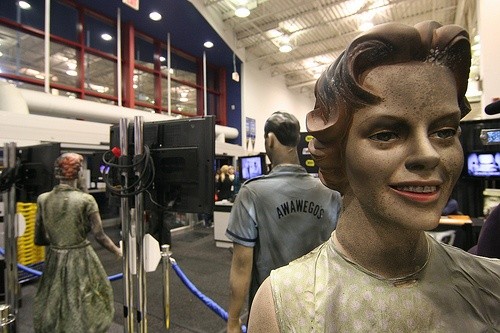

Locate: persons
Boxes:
[224,111,344,333]
[205,165,239,229]
[243,18,500,333]
[31,153,126,333]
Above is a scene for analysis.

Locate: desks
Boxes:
[439,215,484,251]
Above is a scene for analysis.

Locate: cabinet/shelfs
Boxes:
[213,205,233,248]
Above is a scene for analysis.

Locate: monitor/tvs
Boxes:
[0,143,61,203]
[466,150,500,177]
[238,155,267,179]
[109,115,215,215]
